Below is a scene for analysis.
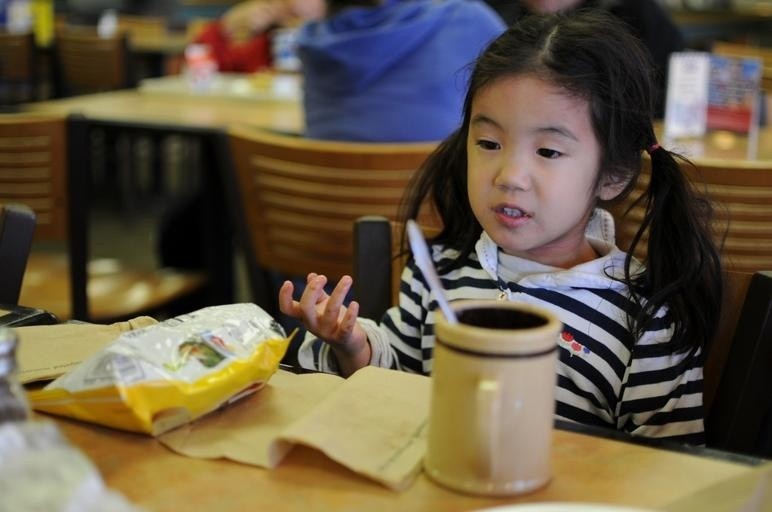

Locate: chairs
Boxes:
[224,124,441,306]
[637,257,771,451]
[66,115,239,326]
[3,115,66,256]
[615,153,771,272]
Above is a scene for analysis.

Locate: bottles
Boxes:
[0,326,35,427]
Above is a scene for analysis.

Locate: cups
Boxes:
[422,300,564,498]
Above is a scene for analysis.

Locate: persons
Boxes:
[487,1,689,123]
[151,2,329,270]
[275,5,724,457]
[293,2,511,152]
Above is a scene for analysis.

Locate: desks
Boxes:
[0,307,770,507]
[1,74,303,325]
